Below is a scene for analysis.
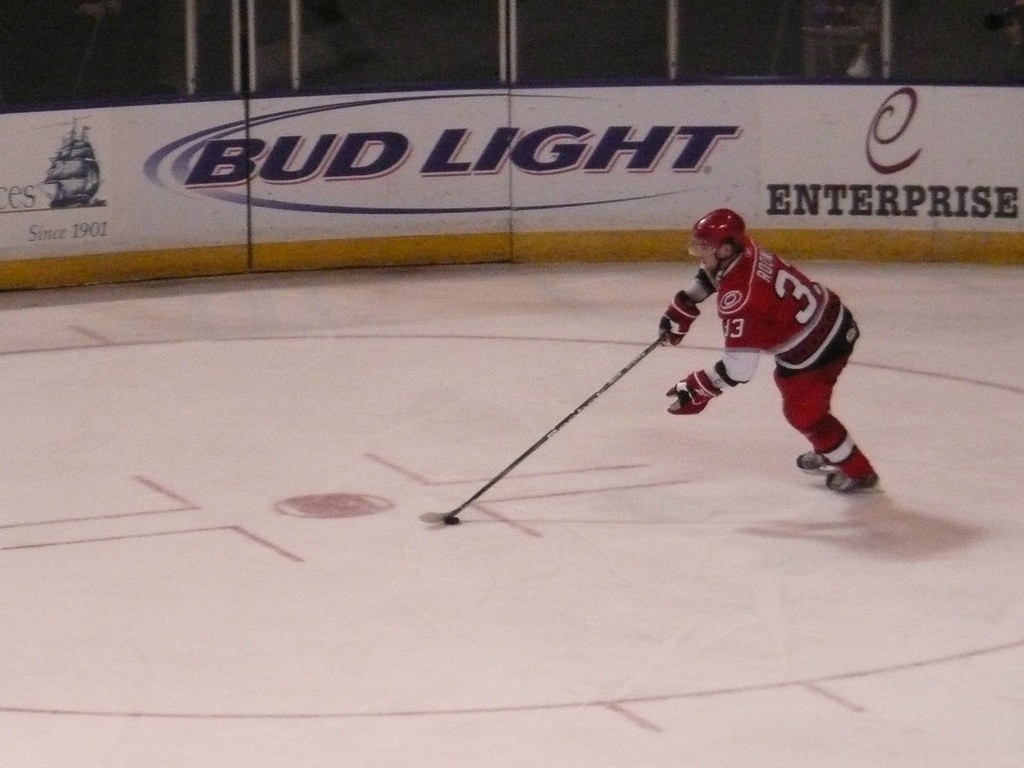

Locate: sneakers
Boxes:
[826,470,884,495]
[796,450,839,476]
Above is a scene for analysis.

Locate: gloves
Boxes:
[658,290,700,345]
[667,370,722,415]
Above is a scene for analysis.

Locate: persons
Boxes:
[658,208,878,493]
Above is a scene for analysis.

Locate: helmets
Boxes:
[686,208,745,259]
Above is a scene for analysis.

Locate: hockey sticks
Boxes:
[418,332,666,525]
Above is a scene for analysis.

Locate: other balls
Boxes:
[443,516,460,525]
